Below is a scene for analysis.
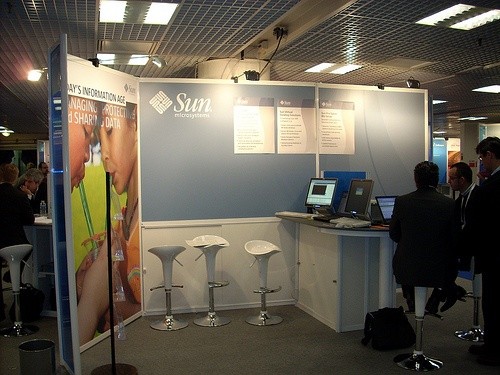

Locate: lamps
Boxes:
[150,55,167,68]
[406,79,421,89]
[26,67,48,81]
[0,129,14,137]
[244,70,260,81]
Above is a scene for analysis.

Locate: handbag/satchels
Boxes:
[359,306,417,352]
[9,282,45,323]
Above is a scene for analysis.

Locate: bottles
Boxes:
[40,199,47,218]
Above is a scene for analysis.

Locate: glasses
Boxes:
[478,156,483,161]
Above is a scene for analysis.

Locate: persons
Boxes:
[39,162,48,203]
[389,161,463,317]
[67,93,139,347]
[469,137,500,363]
[440,162,479,312]
[0,165,35,320]
[19,168,43,215]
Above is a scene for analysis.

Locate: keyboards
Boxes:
[331,217,371,228]
[276,212,314,218]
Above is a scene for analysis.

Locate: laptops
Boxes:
[375,195,398,224]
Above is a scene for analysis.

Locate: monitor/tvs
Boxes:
[345,178,374,215]
[305,178,338,208]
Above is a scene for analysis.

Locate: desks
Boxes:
[274,211,393,334]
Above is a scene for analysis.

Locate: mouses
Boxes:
[335,223,344,228]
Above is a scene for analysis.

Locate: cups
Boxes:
[80,231,127,303]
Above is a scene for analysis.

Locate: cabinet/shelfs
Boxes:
[23,214,57,317]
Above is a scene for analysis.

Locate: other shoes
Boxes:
[2,272,27,288]
[439,286,467,312]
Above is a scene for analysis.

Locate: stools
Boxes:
[147,245,189,331]
[245,240,283,327]
[193,235,231,327]
[454,256,484,342]
[0,244,39,338]
[393,286,443,371]
